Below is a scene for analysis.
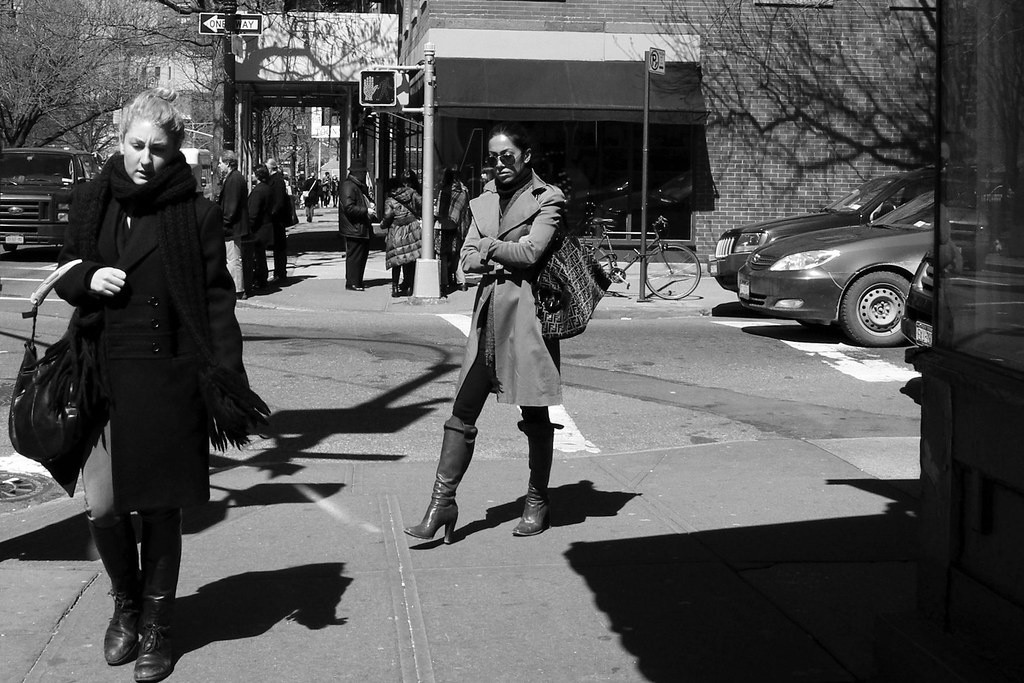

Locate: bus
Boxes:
[179,147,212,192]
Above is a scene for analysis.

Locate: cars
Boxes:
[899,243,936,353]
[707,164,931,293]
[737,190,933,348]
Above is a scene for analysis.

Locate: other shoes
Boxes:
[345,283,363,291]
[400,285,411,296]
[234,291,248,300]
[359,282,368,287]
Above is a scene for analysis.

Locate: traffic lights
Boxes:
[359,70,397,106]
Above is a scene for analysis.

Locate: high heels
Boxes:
[442,284,450,293]
[391,287,400,298]
[458,282,468,292]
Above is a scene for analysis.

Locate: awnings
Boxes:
[433,56,709,126]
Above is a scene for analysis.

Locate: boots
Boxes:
[403,416,478,544]
[85,507,141,666]
[511,421,563,537]
[134,508,182,682]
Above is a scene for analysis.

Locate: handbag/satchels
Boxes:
[281,195,299,226]
[8,259,106,466]
[528,183,613,341]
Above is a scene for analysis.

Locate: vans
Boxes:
[0,147,99,258]
[280,161,291,178]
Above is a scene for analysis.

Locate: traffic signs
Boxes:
[198,12,264,36]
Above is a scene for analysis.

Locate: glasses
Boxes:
[486,145,529,167]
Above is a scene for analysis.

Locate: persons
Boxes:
[338,158,377,291]
[321,171,340,209]
[481,167,497,183]
[216,150,271,300]
[404,120,564,545]
[266,158,290,287]
[53,86,271,682]
[433,166,472,299]
[380,177,422,297]
[302,172,320,223]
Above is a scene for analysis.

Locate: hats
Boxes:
[346,159,371,172]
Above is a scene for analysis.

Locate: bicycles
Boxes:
[578,215,702,301]
